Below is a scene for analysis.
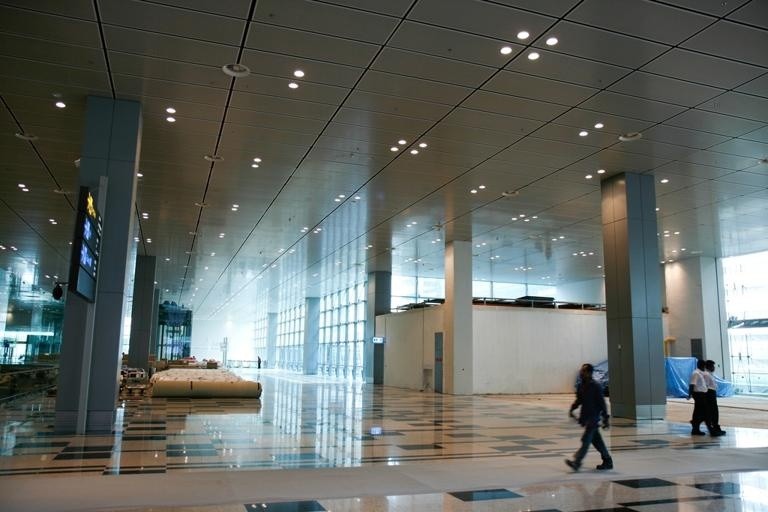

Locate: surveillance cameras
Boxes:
[52,286,62,300]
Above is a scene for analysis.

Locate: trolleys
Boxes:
[121,366,149,394]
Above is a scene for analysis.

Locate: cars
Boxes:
[574,358,609,398]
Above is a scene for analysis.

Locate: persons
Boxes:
[563,363,614,473]
[686,359,721,437]
[689,360,727,435]
[257,356,261,368]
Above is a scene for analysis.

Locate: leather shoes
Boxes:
[692,424,726,436]
[567,460,581,471]
[597,460,613,469]
